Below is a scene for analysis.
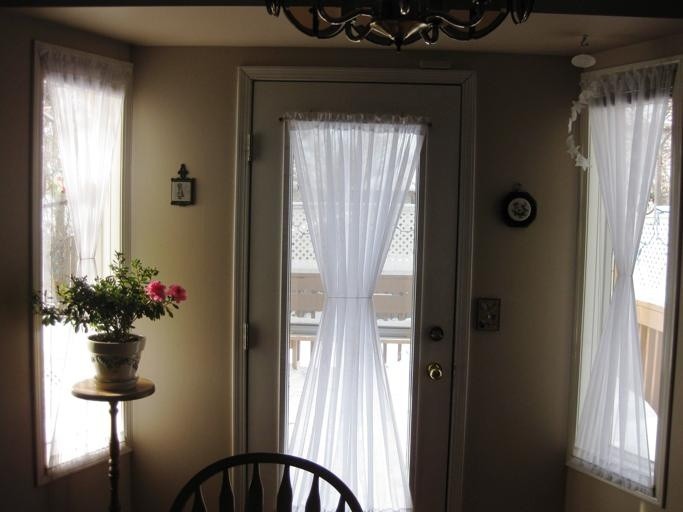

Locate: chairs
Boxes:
[170,453,363,512]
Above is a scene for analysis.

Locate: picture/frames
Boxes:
[170,178,196,206]
[502,192,538,228]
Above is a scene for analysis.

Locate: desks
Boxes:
[72,376,155,512]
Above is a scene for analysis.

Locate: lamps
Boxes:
[265,0,535,53]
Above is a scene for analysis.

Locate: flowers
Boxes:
[30,250,188,342]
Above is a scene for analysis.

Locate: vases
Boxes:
[88,333,146,392]
